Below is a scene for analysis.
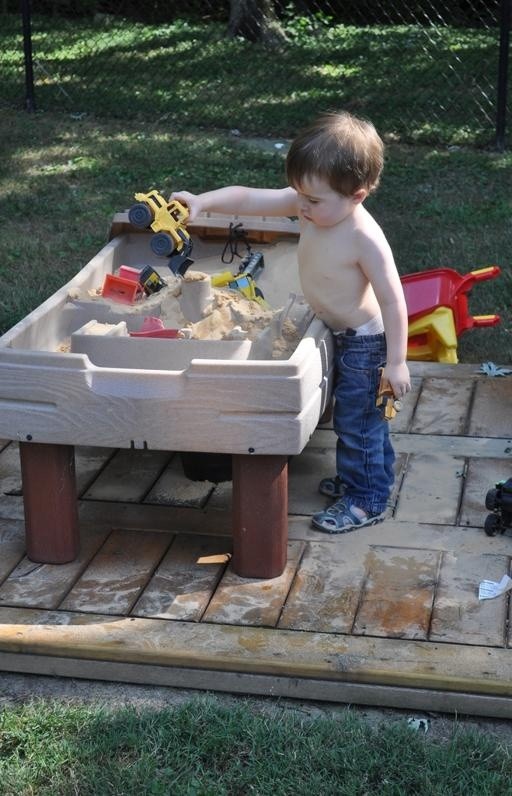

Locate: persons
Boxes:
[168,110,415,533]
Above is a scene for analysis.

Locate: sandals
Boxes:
[319,474,395,498]
[310,498,387,534]
